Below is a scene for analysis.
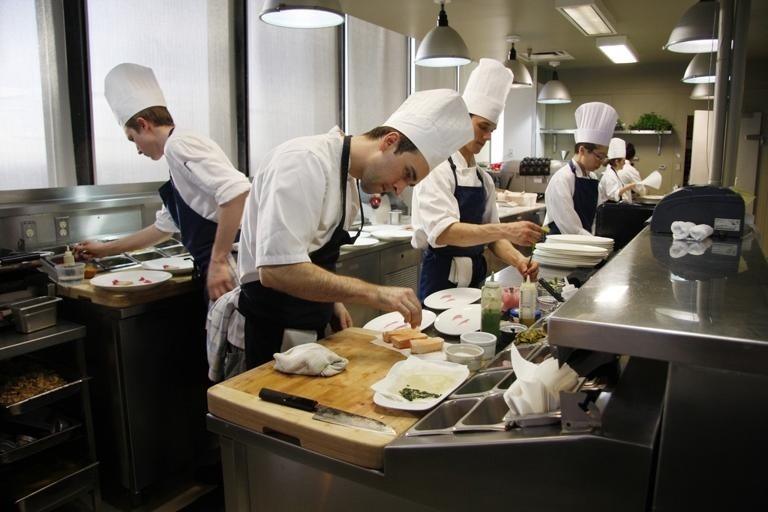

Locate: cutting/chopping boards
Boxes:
[205,325,470,471]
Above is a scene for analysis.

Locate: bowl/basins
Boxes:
[443,331,498,371]
[55,263,85,280]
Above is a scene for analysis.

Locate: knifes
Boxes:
[256,387,397,437]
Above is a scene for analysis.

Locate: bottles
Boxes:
[63,245,75,266]
[518,273,536,323]
[480,270,501,337]
[560,276,579,301]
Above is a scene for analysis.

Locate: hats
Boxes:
[461,58,514,126]
[385,90,476,171]
[607,138,627,160]
[104,63,168,129]
[573,102,619,146]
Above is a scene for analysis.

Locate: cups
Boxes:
[389,209,402,225]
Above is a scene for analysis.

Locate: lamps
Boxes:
[413,0,472,68]
[257,0,346,29]
[502,35,533,88]
[553,0,618,39]
[595,35,641,68]
[537,61,572,104]
[659,0,736,101]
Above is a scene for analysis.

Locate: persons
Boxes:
[590,158,638,237]
[616,142,646,204]
[410,114,543,311]
[541,143,620,236]
[236,126,428,371]
[67,106,253,489]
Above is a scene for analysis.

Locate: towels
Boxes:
[270,341,350,377]
[689,223,715,241]
[448,257,473,288]
[688,238,713,256]
[670,220,696,241]
[669,240,688,259]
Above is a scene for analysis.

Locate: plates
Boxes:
[532,233,615,268]
[363,286,482,335]
[335,224,414,251]
[373,359,470,411]
[89,257,196,291]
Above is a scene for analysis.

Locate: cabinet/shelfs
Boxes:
[0,323,104,512]
